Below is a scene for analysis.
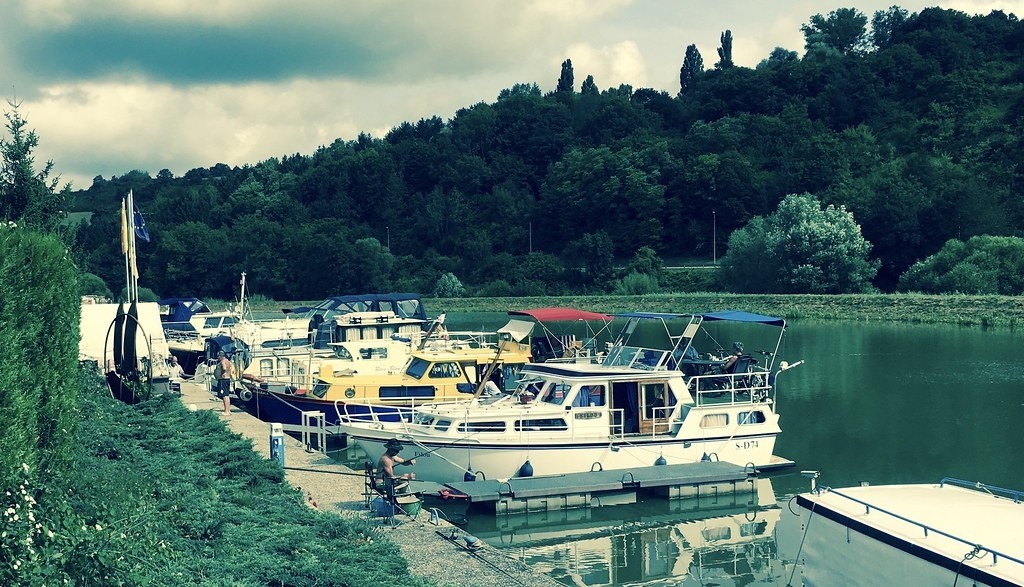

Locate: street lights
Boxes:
[387,227,389,247]
[712,210,716,264]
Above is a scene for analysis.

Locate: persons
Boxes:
[673,337,699,389]
[375,439,416,496]
[704,342,744,398]
[483,374,501,395]
[214,350,232,416]
[168,356,185,378]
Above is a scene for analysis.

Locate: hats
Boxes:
[733,341,744,353]
[674,335,693,347]
[383,438,404,450]
[172,356,178,362]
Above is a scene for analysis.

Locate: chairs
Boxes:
[364,461,402,514]
[381,474,426,529]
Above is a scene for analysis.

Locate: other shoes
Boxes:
[219,411,232,416]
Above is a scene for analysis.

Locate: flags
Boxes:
[122,200,150,279]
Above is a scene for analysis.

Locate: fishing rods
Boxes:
[435,531,526,587]
[392,418,513,467]
[696,321,728,355]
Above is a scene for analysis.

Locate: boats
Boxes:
[475,476,783,587]
[158,279,614,434]
[335,307,805,483]
